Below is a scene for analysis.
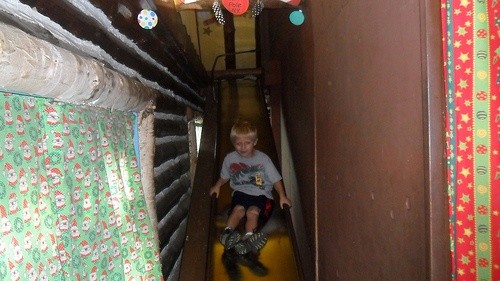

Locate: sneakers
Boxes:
[225,231,268,254]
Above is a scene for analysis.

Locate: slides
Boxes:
[177,69,314,281]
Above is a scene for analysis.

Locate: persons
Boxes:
[208,120,292,255]
